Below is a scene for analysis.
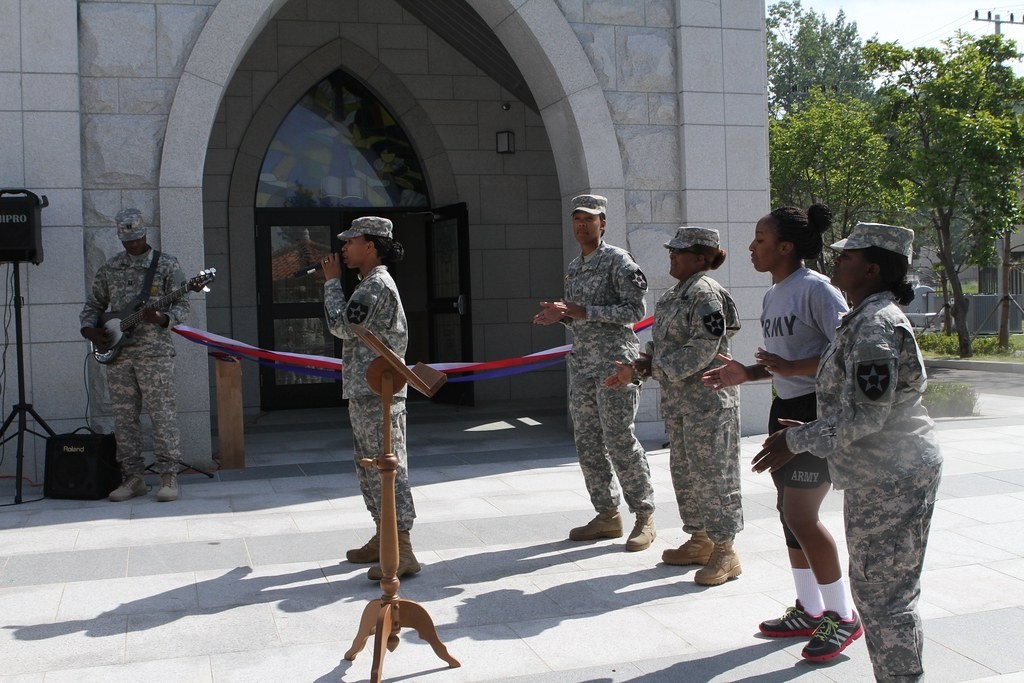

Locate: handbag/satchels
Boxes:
[43,427,122,499]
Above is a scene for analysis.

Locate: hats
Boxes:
[570,194,608,215]
[830,222,914,256]
[663,226,720,249]
[336,216,393,242]
[116,208,145,241]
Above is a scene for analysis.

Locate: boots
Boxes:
[347,524,380,563]
[156,472,178,501]
[367,532,420,580]
[626,513,656,552]
[569,508,624,541]
[694,540,742,585]
[662,530,715,566]
[109,473,148,501]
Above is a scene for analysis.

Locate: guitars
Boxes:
[89,267,217,365]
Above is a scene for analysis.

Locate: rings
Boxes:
[325,260,328,263]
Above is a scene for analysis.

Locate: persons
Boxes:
[605,226,744,583]
[751,221,944,683]
[78,208,192,500]
[321,216,422,580]
[533,194,657,551]
[272,227,325,384]
[700,202,864,660]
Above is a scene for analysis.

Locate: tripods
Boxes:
[0,263,57,504]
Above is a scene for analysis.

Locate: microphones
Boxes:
[293,251,345,279]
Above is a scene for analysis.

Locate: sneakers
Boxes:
[759,599,824,637]
[802,608,864,662]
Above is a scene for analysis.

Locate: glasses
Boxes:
[669,247,700,255]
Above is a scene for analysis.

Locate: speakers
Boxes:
[43,432,122,500]
[0,189,44,266]
